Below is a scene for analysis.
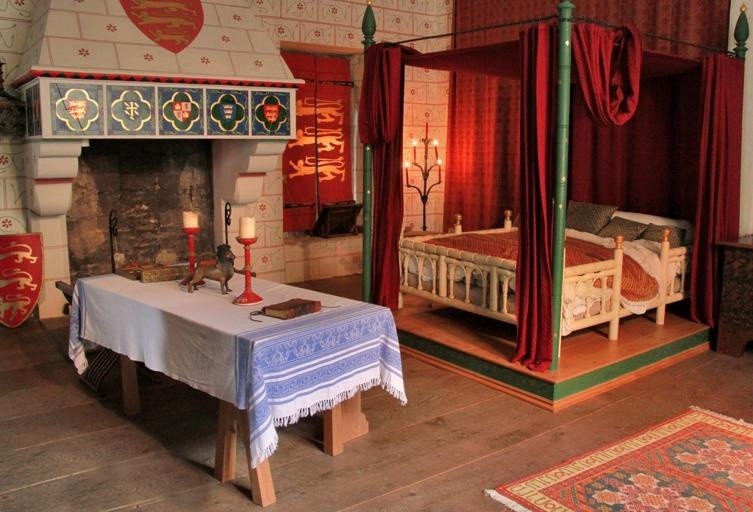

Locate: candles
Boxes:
[183,211,197,227]
[239,217,256,239]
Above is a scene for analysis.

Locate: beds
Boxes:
[397,200,694,357]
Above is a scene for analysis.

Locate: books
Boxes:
[263,297,321,320]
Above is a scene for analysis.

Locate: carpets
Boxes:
[485,405,753,512]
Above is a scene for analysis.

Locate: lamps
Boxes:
[404,123,442,231]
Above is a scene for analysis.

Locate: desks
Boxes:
[68,264,407,506]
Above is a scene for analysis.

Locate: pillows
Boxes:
[566,200,617,234]
[597,217,648,242]
[644,223,686,249]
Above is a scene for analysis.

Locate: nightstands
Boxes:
[716,235,753,357]
